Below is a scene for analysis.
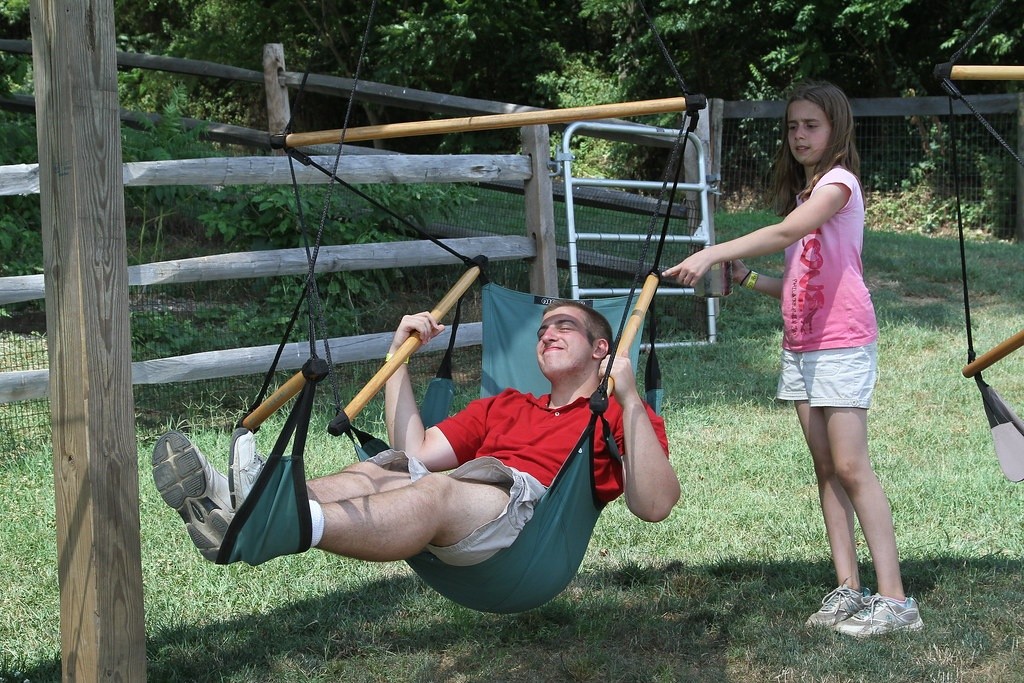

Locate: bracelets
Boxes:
[745,271,759,289]
[738,270,751,286]
[384,353,409,365]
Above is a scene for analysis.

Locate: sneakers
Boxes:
[835,592,924,637]
[152,430,237,563]
[806,586,870,628]
[228,427,264,513]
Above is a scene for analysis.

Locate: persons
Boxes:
[662,81,924,638]
[150,302,681,567]
